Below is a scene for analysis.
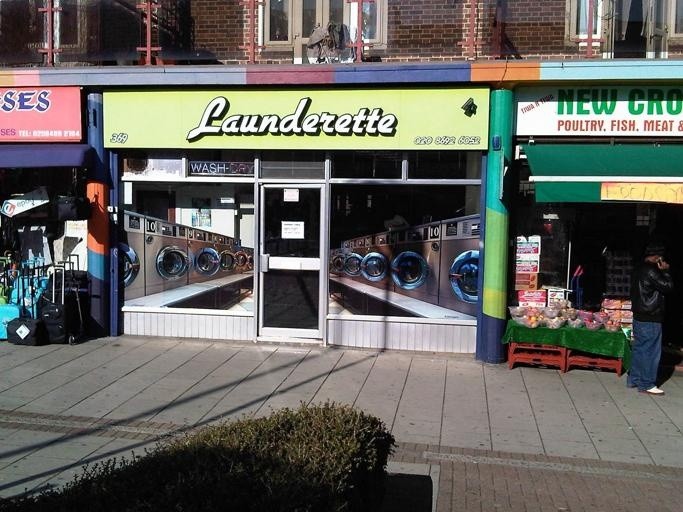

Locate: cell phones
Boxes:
[656,259,661,266]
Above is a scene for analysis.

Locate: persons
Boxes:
[626,238,674,396]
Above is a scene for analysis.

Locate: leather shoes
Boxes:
[627,383,664,396]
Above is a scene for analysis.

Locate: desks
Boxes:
[566,326,632,376]
[508,320,566,372]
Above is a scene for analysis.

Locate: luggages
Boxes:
[0,250,87,346]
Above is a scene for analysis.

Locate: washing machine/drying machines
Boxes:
[328,213,481,319]
[121,211,254,302]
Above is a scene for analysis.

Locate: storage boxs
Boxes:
[512,234,565,308]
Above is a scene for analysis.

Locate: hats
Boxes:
[645,243,664,255]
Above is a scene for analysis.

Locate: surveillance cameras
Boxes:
[461,98,474,111]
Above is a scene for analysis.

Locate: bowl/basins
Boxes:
[510,305,623,329]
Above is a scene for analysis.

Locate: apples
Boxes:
[526,316,537,328]
[607,320,621,326]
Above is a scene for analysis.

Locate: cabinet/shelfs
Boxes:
[605,254,633,296]
